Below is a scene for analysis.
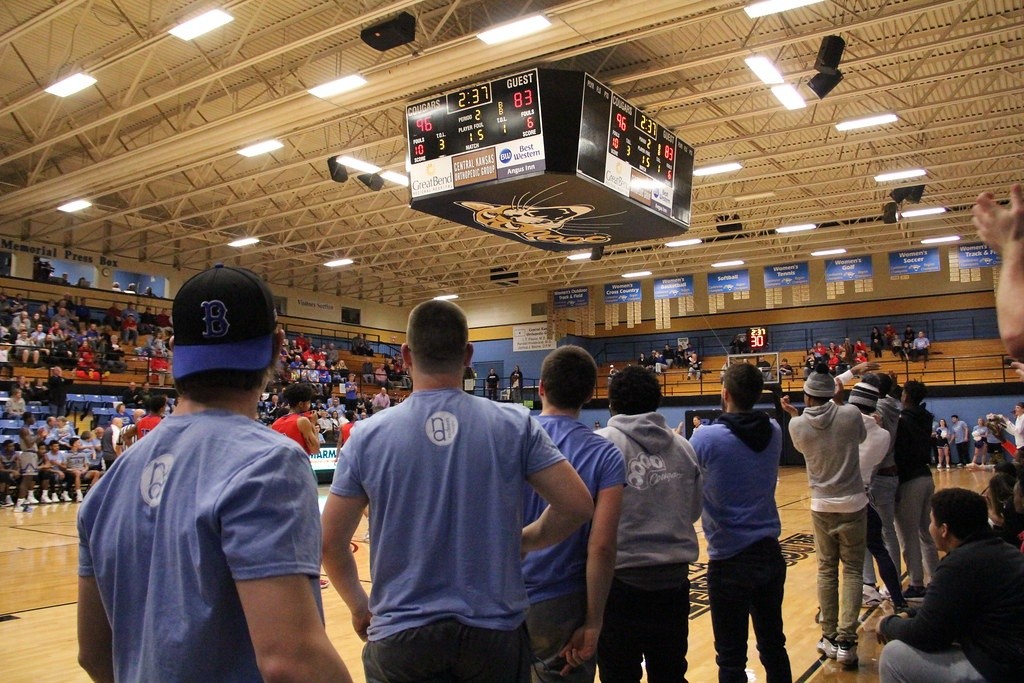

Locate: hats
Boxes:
[172,263,276,379]
[803,363,835,398]
[848,373,881,413]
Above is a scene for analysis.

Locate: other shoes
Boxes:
[926,461,997,470]
[365,532,369,543]
[319,579,330,588]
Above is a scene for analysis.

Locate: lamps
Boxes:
[665,238,702,247]
[168,8,234,41]
[327,155,409,191]
[836,111,899,130]
[322,259,353,267]
[920,235,960,244]
[307,72,367,99]
[711,260,744,268]
[745,35,845,111]
[811,247,847,256]
[777,223,816,234]
[734,190,776,201]
[476,12,552,45]
[44,72,97,97]
[873,169,927,182]
[228,237,260,248]
[744,0,823,19]
[900,205,945,217]
[58,199,93,212]
[622,270,652,278]
[884,202,898,224]
[236,138,284,158]
[890,184,925,204]
[566,247,606,260]
[694,161,742,176]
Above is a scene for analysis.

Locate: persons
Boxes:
[674,362,793,683]
[874,488,1024,683]
[930,402,1024,469]
[971,183,1024,365]
[61,272,71,284]
[718,321,936,384]
[484,368,500,401]
[526,344,626,683]
[0,293,177,510]
[693,416,704,436]
[594,367,704,683]
[320,300,594,683]
[76,262,352,683]
[983,463,1024,554]
[74,277,91,288]
[463,366,477,396]
[607,341,702,387]
[780,362,939,665]
[113,282,163,298]
[509,365,524,404]
[254,327,413,443]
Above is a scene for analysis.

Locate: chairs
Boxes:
[500,387,513,400]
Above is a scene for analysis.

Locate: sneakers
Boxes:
[837,639,858,665]
[862,588,881,607]
[816,635,838,659]
[902,585,926,603]
[0,491,83,512]
[879,586,893,602]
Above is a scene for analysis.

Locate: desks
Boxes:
[523,386,539,388]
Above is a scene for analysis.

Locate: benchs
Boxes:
[589,338,1024,399]
[0,323,415,494]
